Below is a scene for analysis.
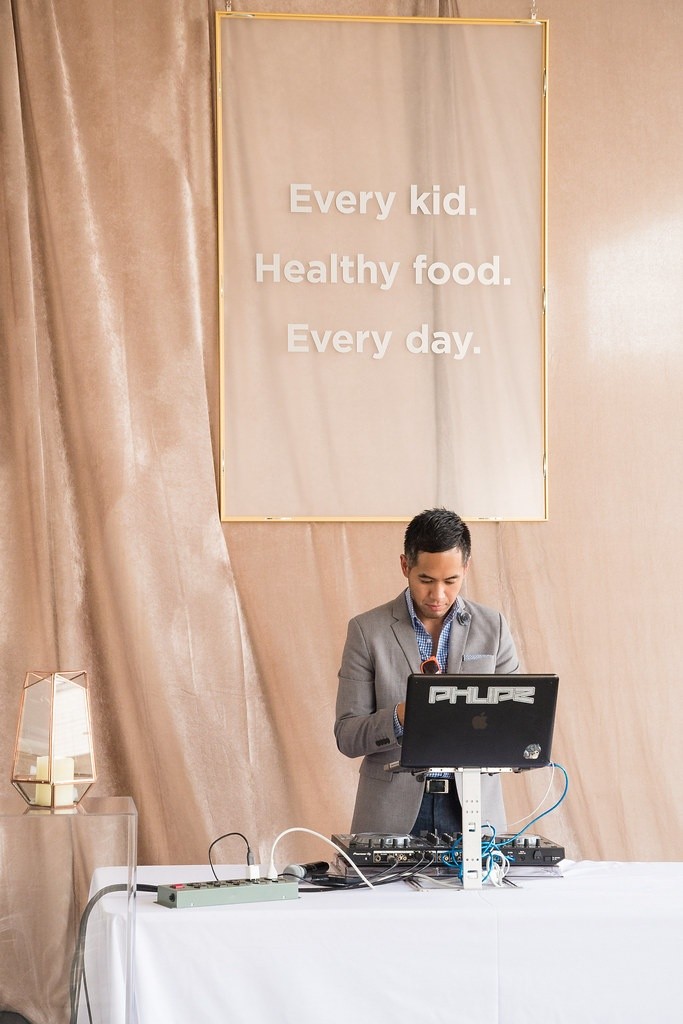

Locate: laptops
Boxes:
[384,674,559,772]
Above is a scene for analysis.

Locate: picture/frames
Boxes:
[214,11,550,525]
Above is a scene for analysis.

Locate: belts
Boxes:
[424,777,457,794]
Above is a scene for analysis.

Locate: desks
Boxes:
[76,860,683,1024]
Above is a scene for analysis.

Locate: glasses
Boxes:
[420,656,440,674]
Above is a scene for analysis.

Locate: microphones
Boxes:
[420,656,442,675]
[282,861,330,880]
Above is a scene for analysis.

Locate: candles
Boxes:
[34,754,76,814]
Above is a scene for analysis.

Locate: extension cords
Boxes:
[157,877,300,910]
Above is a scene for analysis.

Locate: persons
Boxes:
[334,506,522,839]
